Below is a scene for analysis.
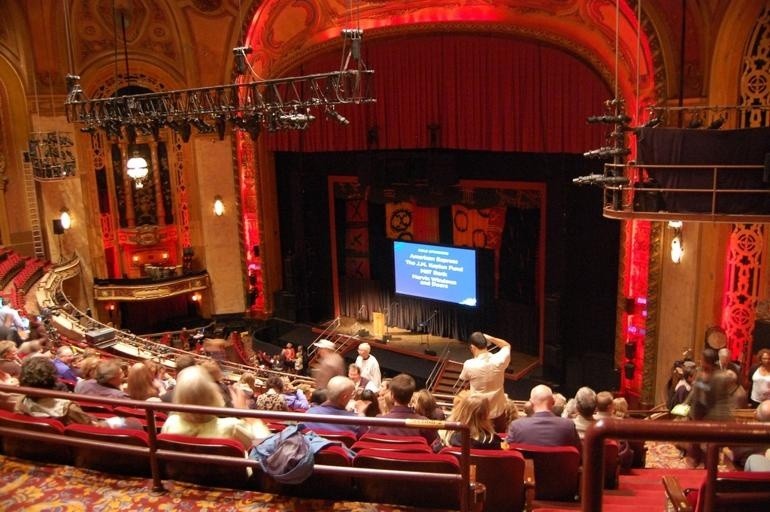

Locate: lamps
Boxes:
[665,222,694,271]
[59,206,76,232]
[125,148,151,192]
[212,194,229,219]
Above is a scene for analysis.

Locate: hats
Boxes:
[313,339,339,351]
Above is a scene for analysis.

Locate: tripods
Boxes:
[57,234,69,266]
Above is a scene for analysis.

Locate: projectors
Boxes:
[85,328,115,344]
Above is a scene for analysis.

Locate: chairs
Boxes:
[56,375,76,389]
[128,417,166,430]
[64,422,152,479]
[510,444,581,498]
[305,425,358,449]
[294,406,309,413]
[87,411,116,421]
[497,429,510,440]
[695,472,770,512]
[314,445,353,500]
[351,440,432,454]
[579,437,620,490]
[0,408,66,465]
[76,400,114,415]
[267,422,287,435]
[437,445,526,512]
[1,240,54,317]
[359,430,427,443]
[351,448,465,508]
[114,403,168,420]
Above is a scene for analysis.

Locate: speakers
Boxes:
[53,219,65,234]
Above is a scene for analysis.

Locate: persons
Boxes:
[674,368,697,404]
[593,391,622,420]
[159,326,206,354]
[0,340,22,377]
[412,389,445,421]
[355,343,382,393]
[123,362,162,405]
[367,374,443,453]
[438,390,504,450]
[523,401,534,417]
[52,345,76,381]
[256,376,289,411]
[75,357,98,381]
[298,375,372,440]
[459,332,511,433]
[613,397,630,418]
[377,377,392,397]
[313,339,346,389]
[76,361,126,400]
[14,357,128,430]
[18,309,62,345]
[307,391,327,407]
[231,372,256,409]
[0,298,24,341]
[567,387,598,439]
[241,333,305,373]
[715,348,741,384]
[281,377,311,410]
[552,393,566,417]
[747,348,770,409]
[161,365,272,449]
[506,384,583,466]
[348,364,369,389]
[202,360,239,418]
[744,449,770,472]
[692,348,726,422]
[161,354,195,403]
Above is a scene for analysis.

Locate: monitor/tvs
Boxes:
[390,239,481,310]
